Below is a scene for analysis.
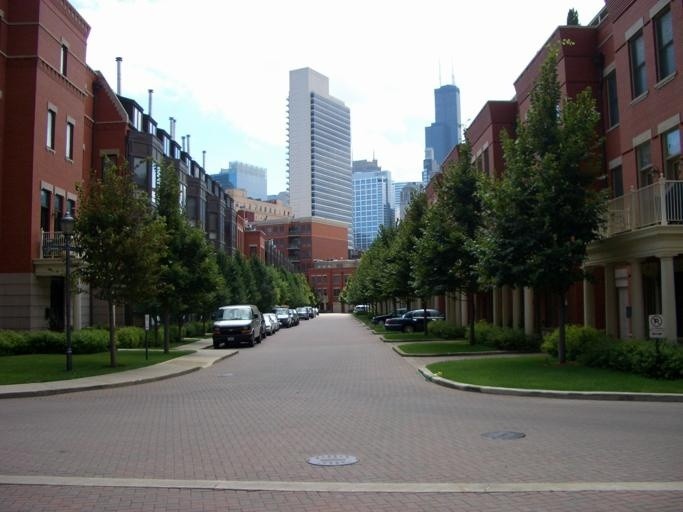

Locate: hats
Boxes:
[262,304,318,336]
[373,307,445,334]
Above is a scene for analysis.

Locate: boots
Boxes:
[213,304,263,347]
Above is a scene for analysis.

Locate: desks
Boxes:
[59,210,76,371]
